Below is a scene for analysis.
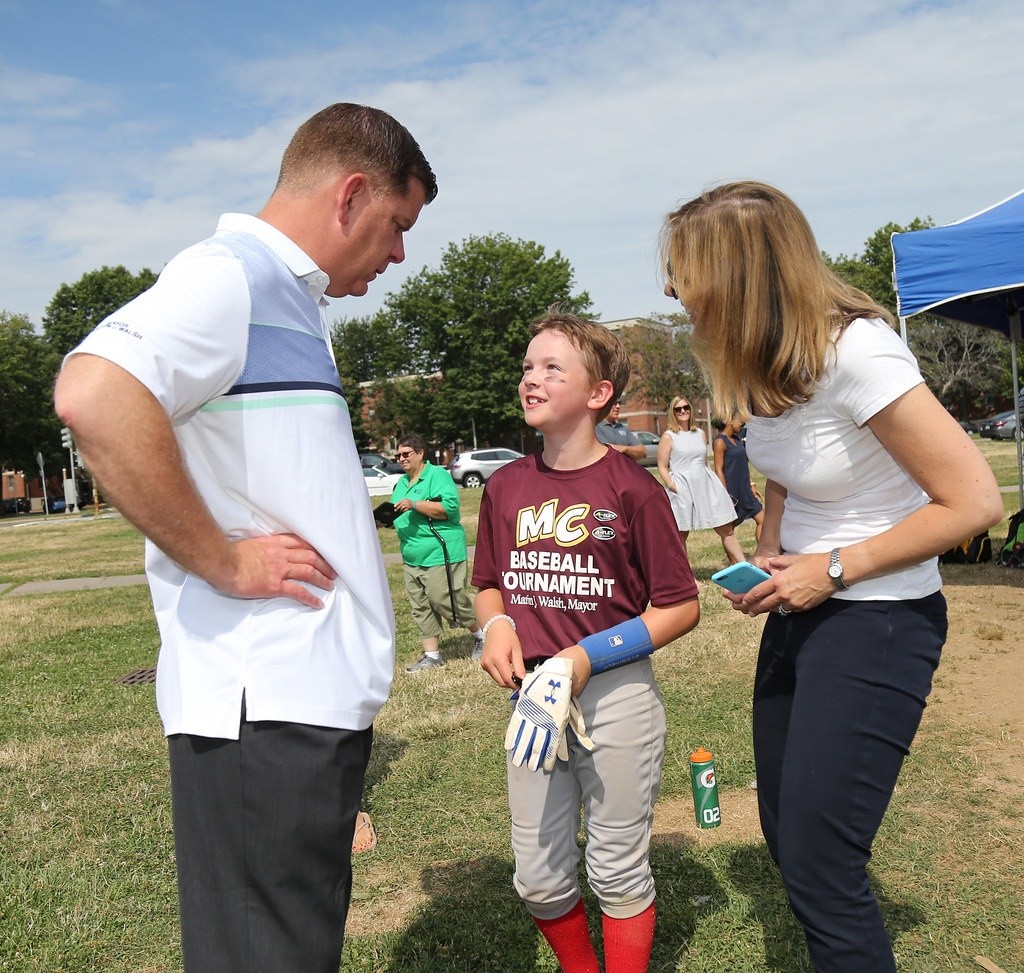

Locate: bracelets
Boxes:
[668,483,673,489]
[481,615,515,644]
[412,499,416,510]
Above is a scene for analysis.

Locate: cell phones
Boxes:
[710,561,772,594]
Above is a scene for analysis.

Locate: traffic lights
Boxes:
[60,427,75,450]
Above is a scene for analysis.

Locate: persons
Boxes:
[54,103,485,973]
[470,312,700,973]
[658,181,1002,973]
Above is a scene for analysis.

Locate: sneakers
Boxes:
[472,636,484,660]
[405,654,445,674]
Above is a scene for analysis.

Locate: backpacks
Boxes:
[995,508,1024,568]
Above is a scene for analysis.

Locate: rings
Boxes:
[779,603,791,616]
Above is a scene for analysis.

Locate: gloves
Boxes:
[504,657,595,773]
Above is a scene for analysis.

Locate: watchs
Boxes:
[827,548,850,591]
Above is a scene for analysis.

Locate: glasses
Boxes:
[674,404,690,413]
[394,450,414,460]
[613,401,621,405]
[741,422,746,428]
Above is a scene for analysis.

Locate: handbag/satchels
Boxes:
[730,494,738,507]
[940,529,992,564]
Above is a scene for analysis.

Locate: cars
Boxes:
[50,495,82,513]
[448,448,527,487]
[978,410,1024,442]
[628,431,661,465]
[359,454,407,496]
[958,421,978,435]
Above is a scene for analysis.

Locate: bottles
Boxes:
[690,747,721,829]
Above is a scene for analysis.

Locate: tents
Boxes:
[891,188,1024,510]
